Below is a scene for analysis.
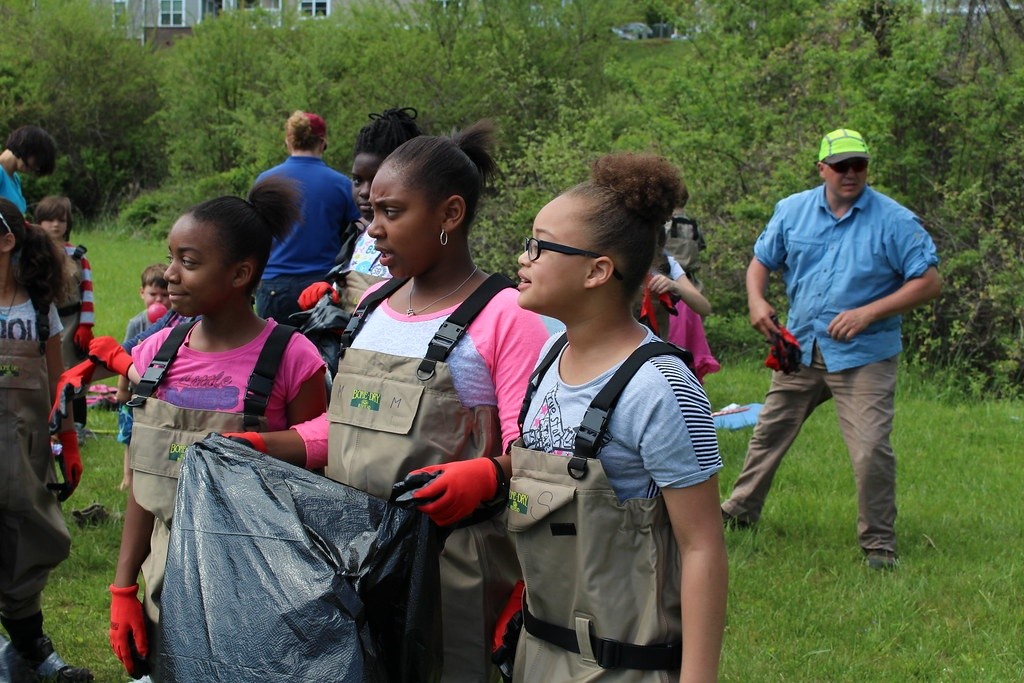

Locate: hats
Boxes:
[818,128,871,165]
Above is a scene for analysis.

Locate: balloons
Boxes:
[147,302,168,324]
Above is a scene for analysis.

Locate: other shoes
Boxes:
[72,422,85,448]
[10,638,95,683]
[865,548,898,572]
[719,506,750,532]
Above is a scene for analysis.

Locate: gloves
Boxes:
[109,584,148,680]
[88,336,135,378]
[764,315,803,374]
[220,430,270,454]
[44,430,85,501]
[49,358,98,431]
[74,324,94,353]
[640,273,679,337]
[490,579,525,668]
[402,457,496,527]
[297,282,340,312]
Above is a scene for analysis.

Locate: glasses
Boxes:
[822,159,869,174]
[524,237,624,281]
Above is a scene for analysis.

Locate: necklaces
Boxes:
[405,264,479,316]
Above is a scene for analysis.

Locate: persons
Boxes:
[717,126,943,573]
[116,262,176,492]
[0,125,58,220]
[0,198,95,683]
[502,146,728,683]
[628,251,712,345]
[249,107,359,326]
[215,112,548,683]
[347,105,422,279]
[31,191,97,448]
[109,166,328,683]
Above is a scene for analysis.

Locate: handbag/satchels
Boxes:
[658,251,721,382]
